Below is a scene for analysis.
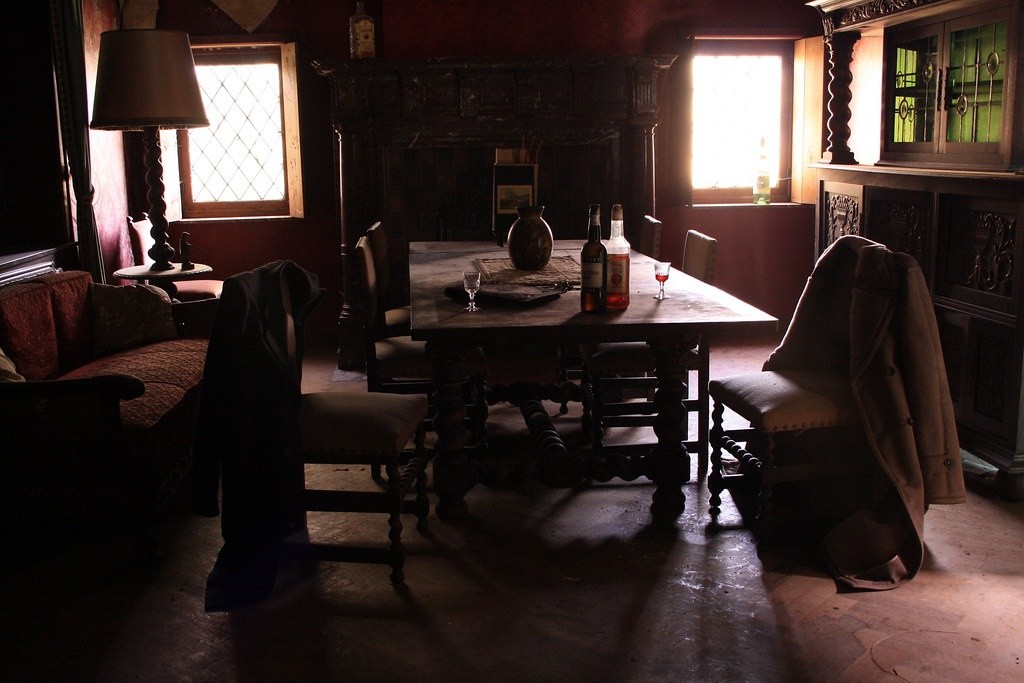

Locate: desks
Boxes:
[114,264,211,334]
[405,240,773,532]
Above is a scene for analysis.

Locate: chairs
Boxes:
[574,215,709,482]
[710,236,966,571]
[203,258,431,616]
[347,220,492,478]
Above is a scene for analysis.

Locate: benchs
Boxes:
[0,267,227,595]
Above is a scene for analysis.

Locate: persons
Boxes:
[181,232,191,266]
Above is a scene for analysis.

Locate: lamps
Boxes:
[89,29,207,270]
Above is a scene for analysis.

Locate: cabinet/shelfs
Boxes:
[813,0,1024,491]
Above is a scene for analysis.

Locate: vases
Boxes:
[508,206,553,269]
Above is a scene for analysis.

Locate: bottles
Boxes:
[606,204,630,309]
[753,138,770,204]
[508,206,553,270]
[581,204,607,312]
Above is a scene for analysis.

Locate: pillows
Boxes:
[88,279,176,359]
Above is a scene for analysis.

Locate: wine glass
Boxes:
[463,271,481,311]
[653,262,671,300]
